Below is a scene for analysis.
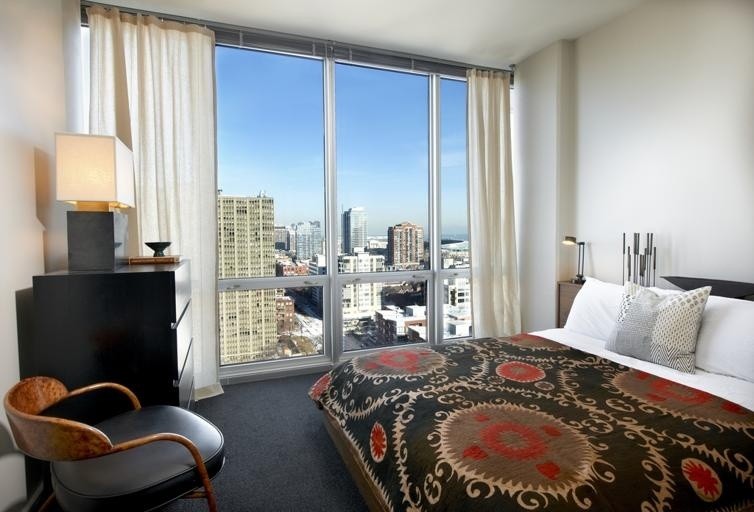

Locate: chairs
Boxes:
[3,373,226,512]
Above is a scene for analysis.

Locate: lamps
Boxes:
[54,132,136,272]
[561,236,585,284]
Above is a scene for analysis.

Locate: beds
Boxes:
[306,277,754,512]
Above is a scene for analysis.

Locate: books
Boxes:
[128,255,182,264]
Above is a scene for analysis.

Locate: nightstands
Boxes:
[558,280,583,330]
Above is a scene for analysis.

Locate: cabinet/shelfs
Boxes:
[31,261,195,412]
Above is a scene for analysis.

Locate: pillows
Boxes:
[564,277,625,341]
[694,296,754,385]
[605,281,712,373]
